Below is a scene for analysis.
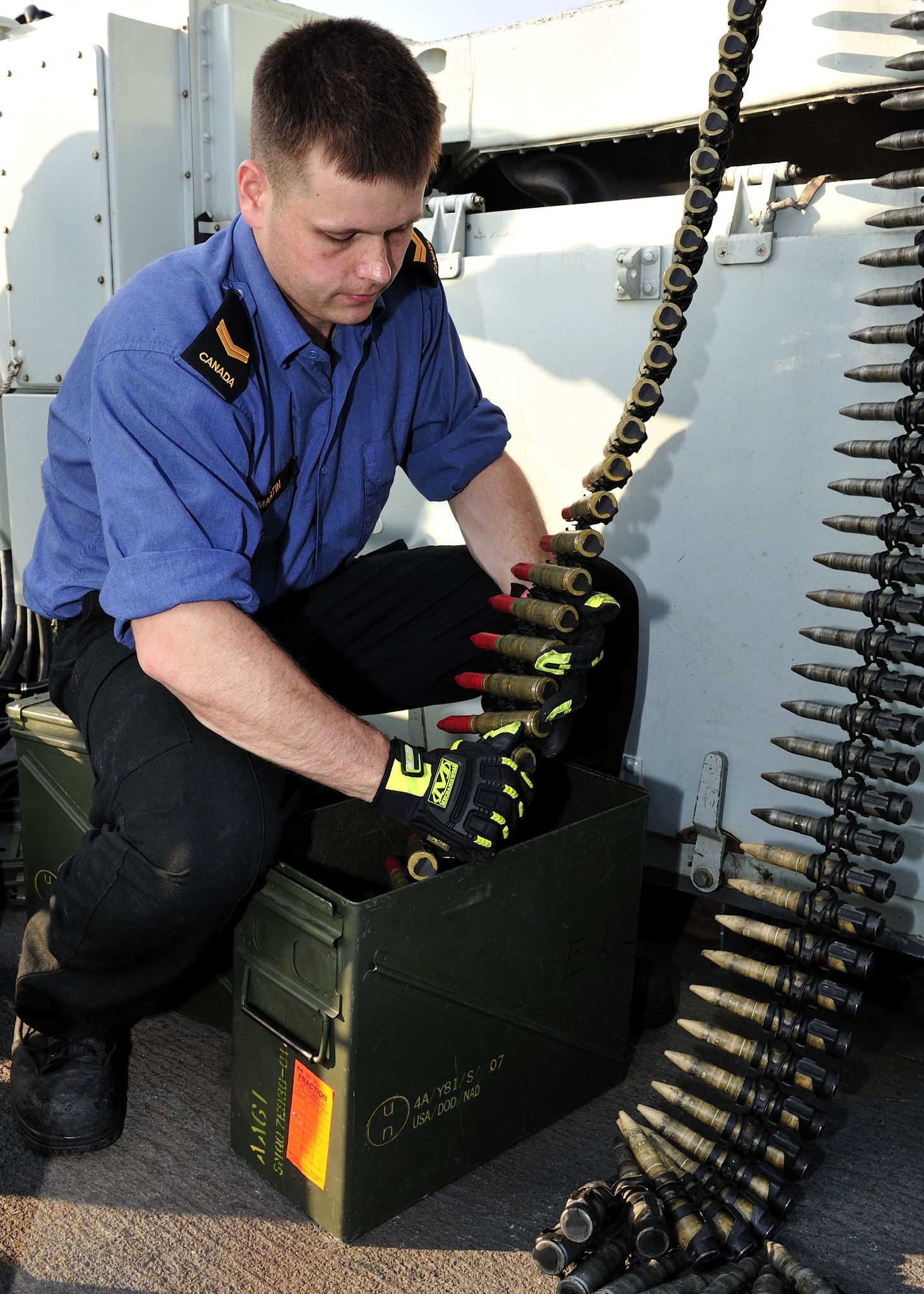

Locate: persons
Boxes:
[14,10,624,1156]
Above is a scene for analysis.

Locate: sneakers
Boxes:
[10,1015,124,1157]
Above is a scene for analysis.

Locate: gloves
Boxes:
[481,581,622,760]
[368,719,537,864]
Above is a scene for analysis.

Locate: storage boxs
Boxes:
[10,691,99,926]
[232,745,653,1250]
